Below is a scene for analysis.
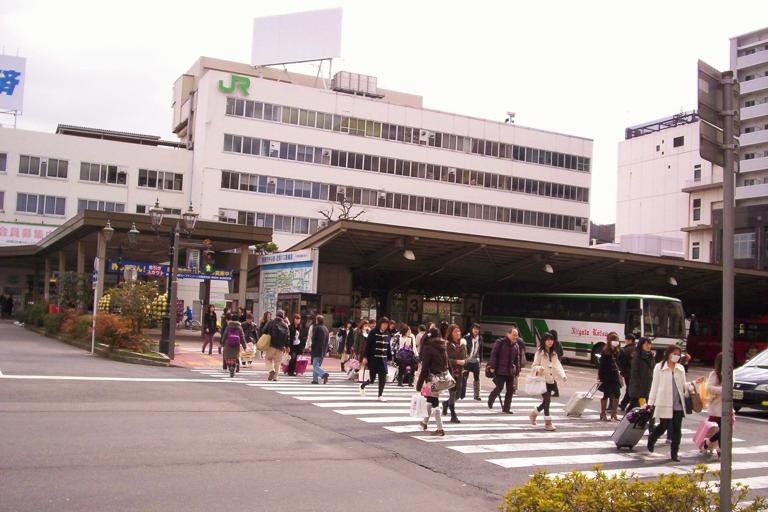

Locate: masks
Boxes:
[612,341,620,348]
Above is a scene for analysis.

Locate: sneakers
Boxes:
[419,421,427,431]
[359,384,365,397]
[378,396,387,402]
[451,418,460,423]
[430,430,443,435]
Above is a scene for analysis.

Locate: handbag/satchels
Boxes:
[626,404,655,424]
[525,376,548,396]
[335,332,343,343]
[485,366,495,377]
[398,349,414,364]
[430,368,456,392]
[344,357,359,380]
[256,334,271,351]
[359,370,371,382]
[685,378,718,414]
[618,371,624,388]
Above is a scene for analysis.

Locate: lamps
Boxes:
[668,276,678,287]
[542,261,555,274]
[401,249,417,262]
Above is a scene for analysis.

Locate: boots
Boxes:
[611,399,621,421]
[544,416,556,430]
[600,399,611,421]
[528,406,541,425]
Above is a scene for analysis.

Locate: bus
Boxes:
[685,311,768,368]
[478,290,697,372]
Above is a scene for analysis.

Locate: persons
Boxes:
[5,294,13,315]
[597,331,735,462]
[184,306,193,327]
[202,306,569,438]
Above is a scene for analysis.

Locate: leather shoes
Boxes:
[323,373,329,384]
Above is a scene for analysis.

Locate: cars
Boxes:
[732,348,768,415]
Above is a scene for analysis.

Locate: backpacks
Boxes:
[228,328,241,347]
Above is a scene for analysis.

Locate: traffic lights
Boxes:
[202,249,216,275]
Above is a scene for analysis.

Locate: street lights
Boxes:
[147,195,200,361]
[102,217,140,288]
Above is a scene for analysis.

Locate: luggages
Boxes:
[294,353,308,375]
[611,413,648,449]
[563,382,602,416]
[693,417,720,450]
